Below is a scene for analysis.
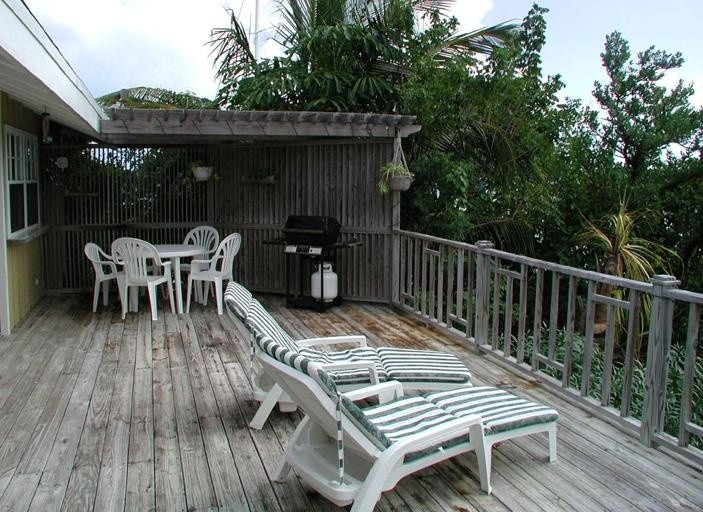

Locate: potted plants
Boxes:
[188,159,214,185]
[377,158,414,194]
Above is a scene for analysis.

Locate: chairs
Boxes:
[172,226,220,303]
[83,242,130,314]
[111,236,175,321]
[223,281,475,430]
[185,232,242,315]
[250,326,561,512]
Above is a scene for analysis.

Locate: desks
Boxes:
[139,244,205,314]
